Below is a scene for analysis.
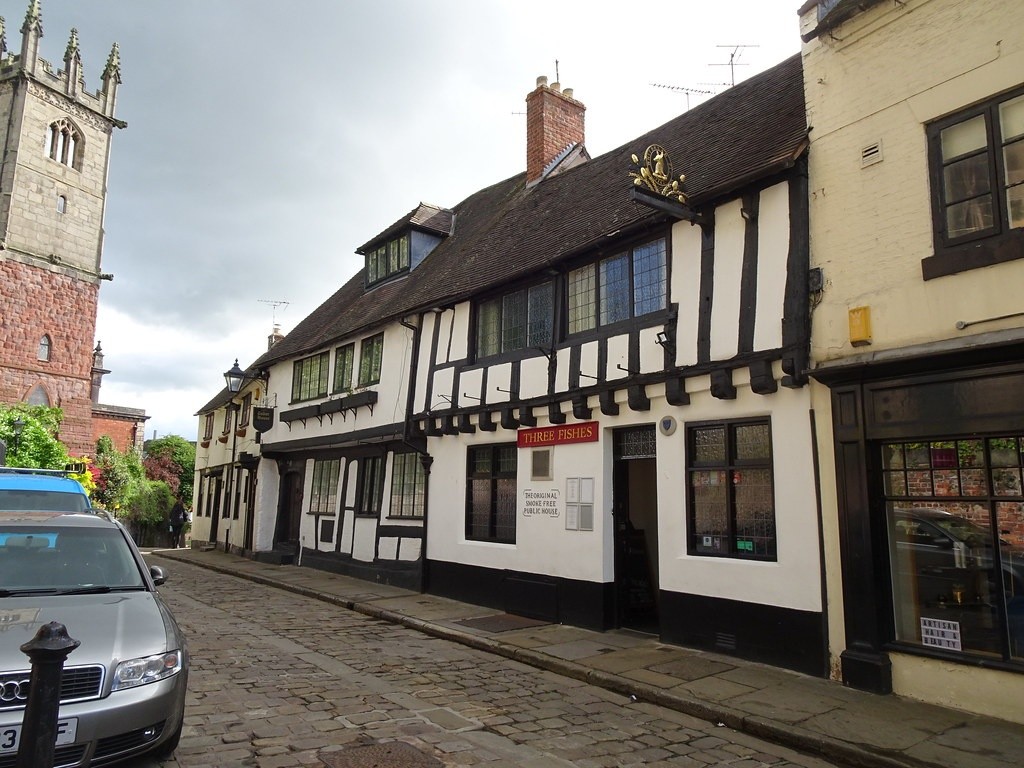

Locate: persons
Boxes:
[186,506,193,523]
[169,501,188,549]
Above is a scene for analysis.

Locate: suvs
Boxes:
[0,465,105,551]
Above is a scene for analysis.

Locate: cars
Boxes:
[0,506,189,768]
[892,508,1024,634]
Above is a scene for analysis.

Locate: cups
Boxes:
[952,582,966,603]
[952,541,966,569]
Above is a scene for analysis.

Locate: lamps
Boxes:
[223,359,270,397]
